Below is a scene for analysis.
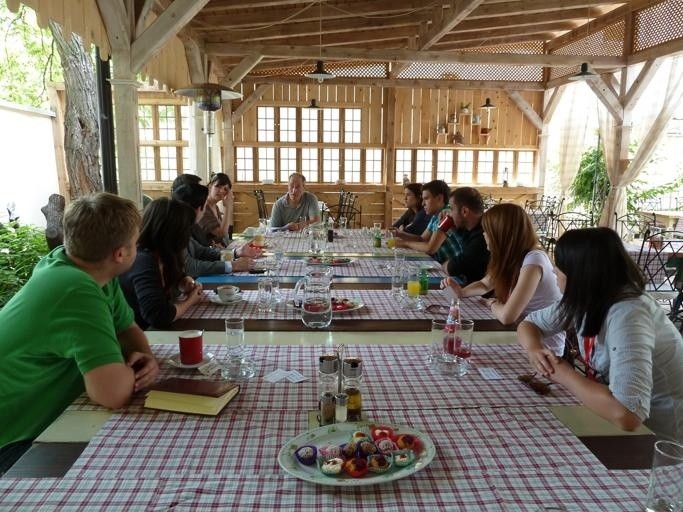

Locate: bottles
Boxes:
[407,267,420,298]
[419,266,428,294]
[326,217,334,243]
[316,391,335,427]
[317,200,325,211]
[440,298,461,362]
[317,356,339,399]
[343,358,362,416]
[374,228,382,248]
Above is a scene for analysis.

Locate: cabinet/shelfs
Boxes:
[437,113,490,147]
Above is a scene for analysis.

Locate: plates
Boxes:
[248,243,269,248]
[287,298,364,313]
[239,226,289,239]
[418,264,436,270]
[279,420,437,485]
[209,296,244,305]
[251,258,268,264]
[302,255,357,264]
[166,353,214,370]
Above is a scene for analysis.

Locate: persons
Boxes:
[172,183,262,277]
[118,197,204,330]
[440,204,567,358]
[172,175,226,249]
[392,183,430,234]
[199,173,233,246]
[396,179,464,264]
[438,186,491,283]
[516,228,682,444]
[0,188,159,470]
[270,172,321,232]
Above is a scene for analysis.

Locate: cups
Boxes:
[268,277,281,305]
[394,248,407,262]
[333,391,350,422]
[429,318,474,378]
[437,216,454,233]
[646,439,683,511]
[256,277,274,313]
[385,228,399,250]
[178,330,203,364]
[339,218,353,235]
[263,247,283,270]
[361,226,368,237]
[248,258,265,270]
[260,252,275,264]
[220,250,234,263]
[217,285,240,301]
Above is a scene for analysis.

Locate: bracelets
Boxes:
[234,250,237,258]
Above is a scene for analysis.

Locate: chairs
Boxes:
[254,190,269,225]
[336,187,359,221]
[481,194,683,336]
[321,204,362,230]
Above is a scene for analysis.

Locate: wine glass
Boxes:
[370,228,375,246]
[263,217,271,237]
[390,267,406,298]
[298,216,306,234]
[220,316,254,379]
[374,223,380,234]
[258,218,265,234]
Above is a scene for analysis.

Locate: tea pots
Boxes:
[301,228,328,254]
[393,292,424,310]
[292,265,333,329]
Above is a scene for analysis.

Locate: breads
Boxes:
[294,445,317,466]
[319,426,420,478]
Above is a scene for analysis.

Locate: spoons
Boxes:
[374,265,386,270]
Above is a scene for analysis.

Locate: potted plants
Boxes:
[460,101,471,113]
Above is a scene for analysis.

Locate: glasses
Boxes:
[425,305,450,315]
[518,372,552,396]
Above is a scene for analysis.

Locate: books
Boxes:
[145,377,241,415]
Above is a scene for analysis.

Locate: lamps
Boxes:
[174,82,244,185]
[567,0,601,81]
[304,59,321,108]
[481,97,496,108]
[304,1,337,85]
